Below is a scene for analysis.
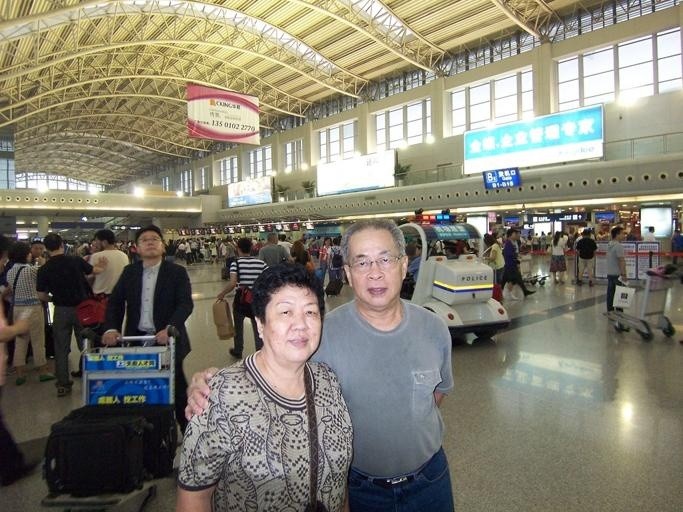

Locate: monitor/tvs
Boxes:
[503,215,520,226]
[595,213,617,225]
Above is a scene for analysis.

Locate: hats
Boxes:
[443,240,457,249]
[415,238,422,248]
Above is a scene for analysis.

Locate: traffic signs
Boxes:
[565,214,584,222]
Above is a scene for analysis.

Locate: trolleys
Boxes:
[35,329,181,509]
[523,272,550,287]
[603,264,677,341]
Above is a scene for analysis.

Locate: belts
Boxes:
[374,476,413,486]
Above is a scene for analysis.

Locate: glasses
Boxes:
[347,254,402,270]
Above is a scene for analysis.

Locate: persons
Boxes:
[165,233,346,359]
[36,233,102,398]
[175,263,354,512]
[0,236,37,487]
[185,222,454,512]
[66,228,141,333]
[1,239,53,356]
[6,245,56,386]
[399,236,478,300]
[483,226,683,319]
[96,225,193,437]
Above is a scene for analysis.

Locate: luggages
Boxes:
[42,404,177,497]
[494,263,504,303]
[220,260,230,279]
[325,279,343,296]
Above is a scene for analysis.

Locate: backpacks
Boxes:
[331,248,343,267]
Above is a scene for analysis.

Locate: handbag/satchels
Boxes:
[238,284,254,305]
[612,284,636,308]
[213,298,235,340]
[307,262,316,274]
[76,293,108,327]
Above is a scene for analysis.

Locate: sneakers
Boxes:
[508,292,518,302]
[555,278,593,287]
[524,290,535,296]
[15,371,81,397]
[229,348,243,358]
